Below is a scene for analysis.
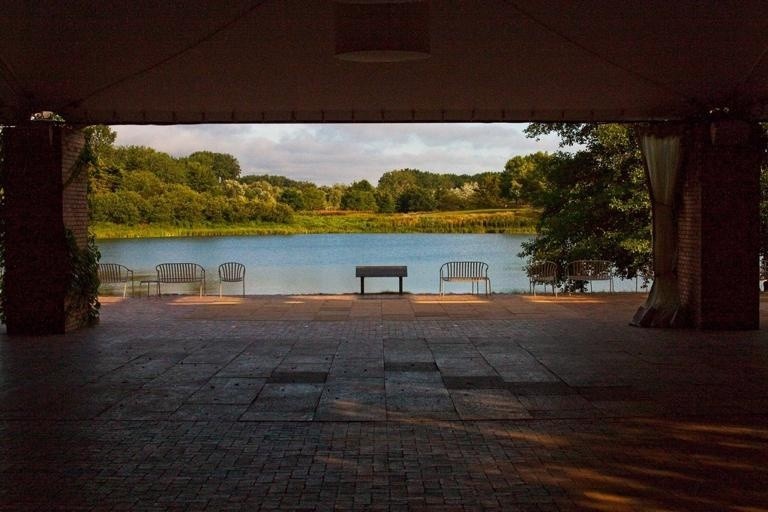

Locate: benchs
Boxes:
[97,262,206,300]
[564,259,615,296]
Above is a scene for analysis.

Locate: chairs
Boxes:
[218,262,246,298]
[526,260,558,299]
[439,261,492,298]
